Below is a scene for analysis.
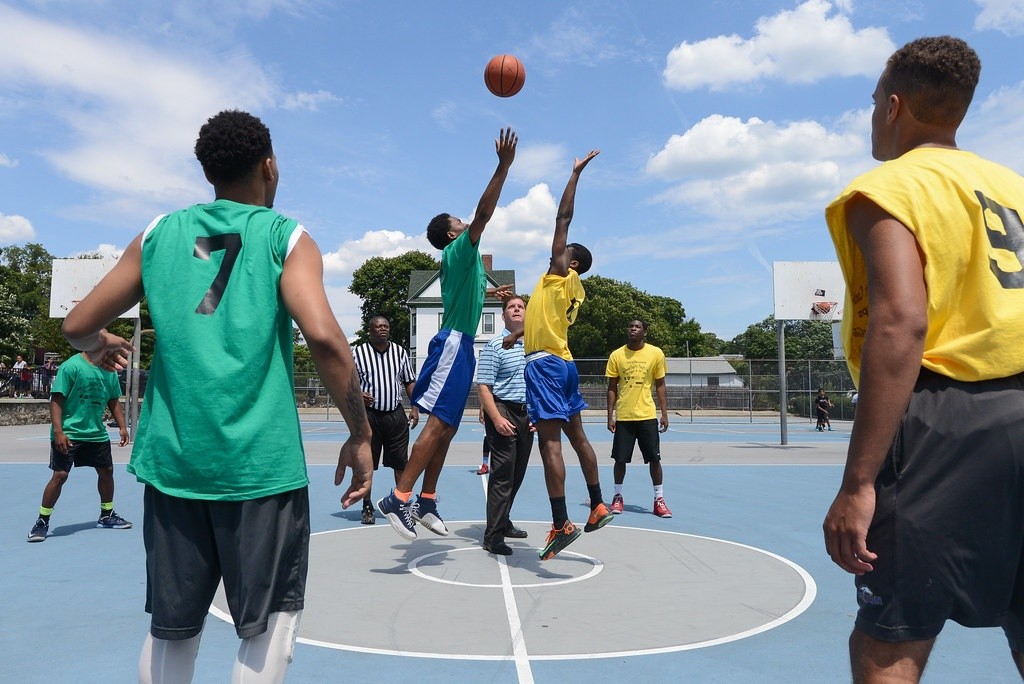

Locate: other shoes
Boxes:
[815,426,819,431]
[819,427,823,432]
[828,427,832,431]
[824,428,826,431]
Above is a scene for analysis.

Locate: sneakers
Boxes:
[410,494,449,536]
[477,464,488,475]
[97,509,133,529]
[654,497,672,518]
[377,488,417,541]
[539,520,581,561]
[361,505,375,523]
[610,493,623,513]
[27,518,49,542]
[584,502,612,532]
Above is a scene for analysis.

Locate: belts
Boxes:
[503,399,526,411]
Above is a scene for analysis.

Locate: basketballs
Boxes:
[484,55,526,98]
[818,302,831,314]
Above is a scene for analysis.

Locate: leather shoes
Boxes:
[505,526,528,538]
[482,541,513,555]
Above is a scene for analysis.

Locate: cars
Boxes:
[32,366,59,400]
[118,369,149,397]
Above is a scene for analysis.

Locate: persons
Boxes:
[376,125,518,541]
[27,329,129,543]
[500,148,615,561]
[474,294,537,554]
[42,357,59,399]
[822,35,1024,684]
[10,356,27,399]
[61,107,374,684]
[815,388,835,432]
[605,317,673,519]
[477,402,490,474]
[351,316,418,526]
